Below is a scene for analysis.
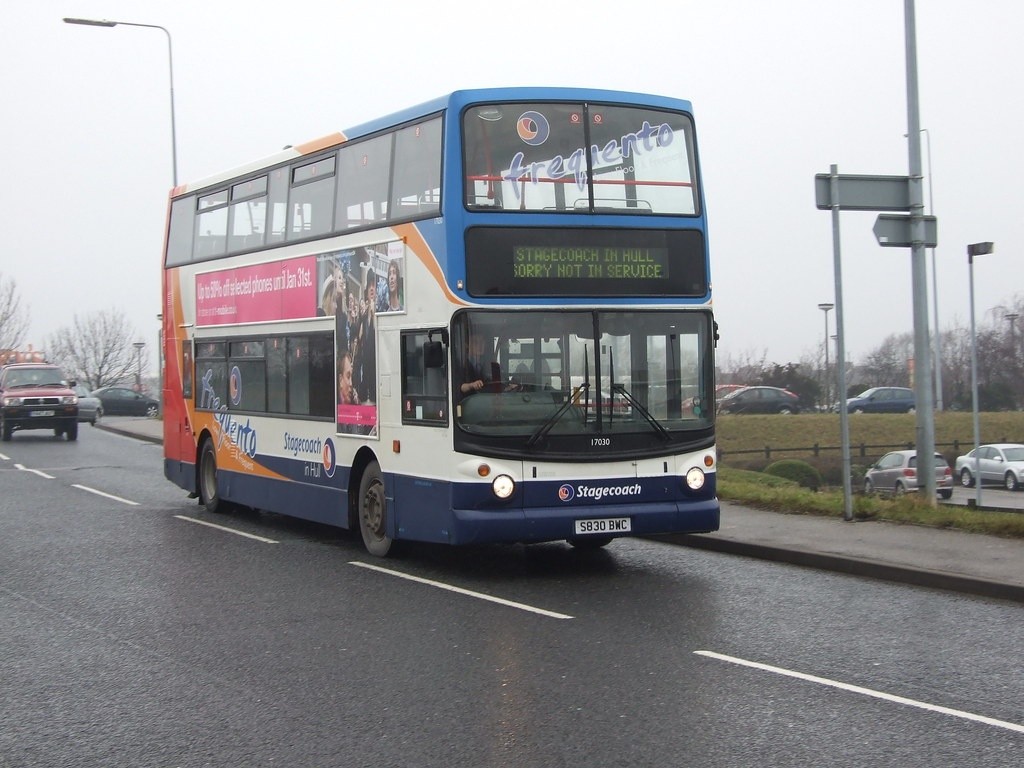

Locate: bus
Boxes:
[161,89,720,559]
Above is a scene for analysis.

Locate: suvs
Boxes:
[0,363,78,441]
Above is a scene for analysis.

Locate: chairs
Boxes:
[426,348,446,412]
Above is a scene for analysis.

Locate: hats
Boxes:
[362,268,380,297]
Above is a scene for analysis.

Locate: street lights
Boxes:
[62,16,177,189]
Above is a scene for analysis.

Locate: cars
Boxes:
[681,384,746,416]
[76,384,104,427]
[955,444,1024,490]
[836,387,916,414]
[94,386,160,417]
[864,450,953,499]
[715,386,799,415]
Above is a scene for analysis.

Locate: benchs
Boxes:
[280,225,311,242]
[243,231,281,248]
[418,195,503,214]
[391,200,439,219]
[573,198,653,214]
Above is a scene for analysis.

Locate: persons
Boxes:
[317,259,404,402]
[450,332,523,400]
[336,349,367,435]
